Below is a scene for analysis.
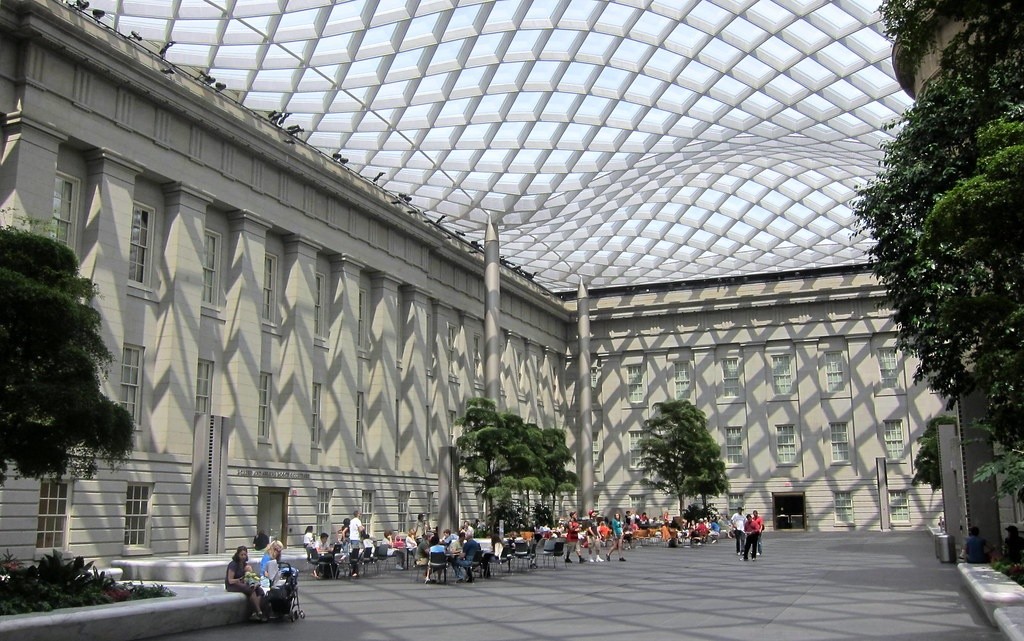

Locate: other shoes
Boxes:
[606,554,610,560]
[738,552,740,556]
[742,552,744,554]
[467,576,474,583]
[249,611,266,620]
[579,559,586,563]
[565,558,572,563]
[619,558,626,561]
[739,558,748,560]
[756,552,761,555]
[456,578,463,582]
[752,557,757,561]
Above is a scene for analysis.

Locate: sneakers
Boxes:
[589,557,594,562]
[424,577,431,584]
[712,539,716,543]
[396,565,403,570]
[352,570,359,577]
[596,555,604,562]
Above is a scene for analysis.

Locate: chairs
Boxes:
[302,516,754,584]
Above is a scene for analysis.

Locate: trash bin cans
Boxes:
[935,533,957,563]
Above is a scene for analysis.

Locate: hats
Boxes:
[704,517,707,520]
[589,509,593,515]
[569,511,576,517]
[700,518,703,522]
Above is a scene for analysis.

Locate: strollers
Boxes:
[261,562,305,622]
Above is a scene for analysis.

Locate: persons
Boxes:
[348,510,365,549]
[730,507,764,561]
[447,532,465,551]
[532,521,609,548]
[564,511,587,563]
[415,532,437,580]
[381,531,406,571]
[464,519,487,539]
[406,527,419,568]
[586,509,605,562]
[335,518,351,562]
[961,524,1024,565]
[224,528,284,620]
[303,525,375,579]
[621,510,735,550]
[606,513,626,562]
[481,535,504,578]
[451,532,481,583]
[415,513,425,545]
[438,529,454,545]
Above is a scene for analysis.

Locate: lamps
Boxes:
[76,0,89,12]
[196,70,539,282]
[127,31,142,41]
[92,9,104,21]
[158,42,174,59]
